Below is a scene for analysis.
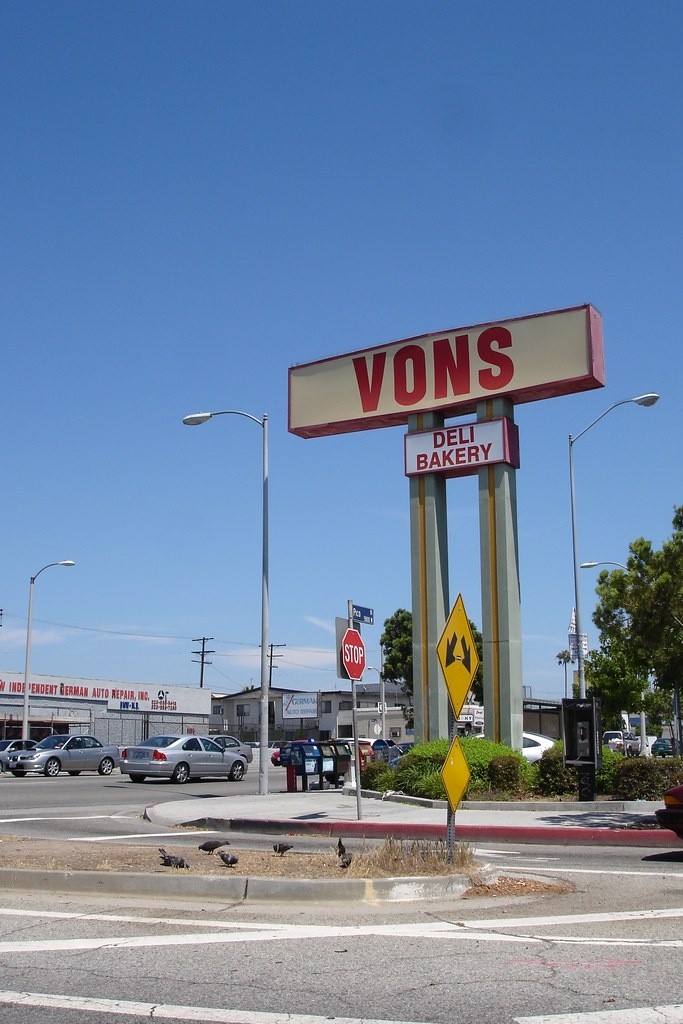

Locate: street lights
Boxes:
[182,410,269,795]
[580,561,649,756]
[21,561,75,740]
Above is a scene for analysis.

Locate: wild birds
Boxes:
[218,850,239,868]
[157,848,191,869]
[198,840,230,856]
[337,837,352,867]
[273,843,294,857]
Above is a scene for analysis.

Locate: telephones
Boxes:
[561,697,602,769]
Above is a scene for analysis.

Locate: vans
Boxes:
[603,730,640,756]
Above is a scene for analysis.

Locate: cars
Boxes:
[0,735,253,783]
[467,730,559,766]
[651,737,681,759]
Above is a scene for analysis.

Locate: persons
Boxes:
[464,722,476,737]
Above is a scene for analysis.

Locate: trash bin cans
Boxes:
[291,739,401,776]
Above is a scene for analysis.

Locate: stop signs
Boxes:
[342,629,367,680]
